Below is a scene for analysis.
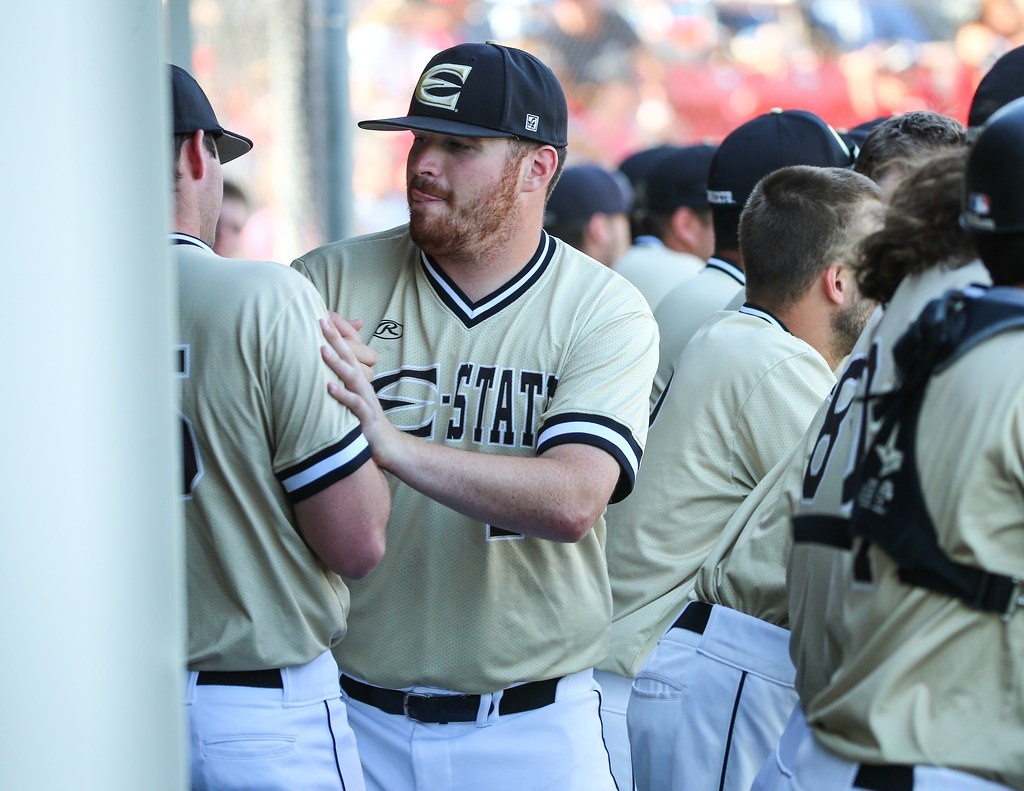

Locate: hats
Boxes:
[971,47,1024,127]
[359,42,567,148]
[535,103,900,230]
[169,63,252,164]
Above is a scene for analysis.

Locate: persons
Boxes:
[545,166,629,263]
[292,40,659,791]
[593,47,1024,791]
[169,62,390,791]
[214,183,247,259]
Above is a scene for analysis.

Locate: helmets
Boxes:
[959,100,1024,234]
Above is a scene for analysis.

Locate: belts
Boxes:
[339,669,560,727]
[855,763,915,791]
[671,603,713,636]
[198,664,283,690]
